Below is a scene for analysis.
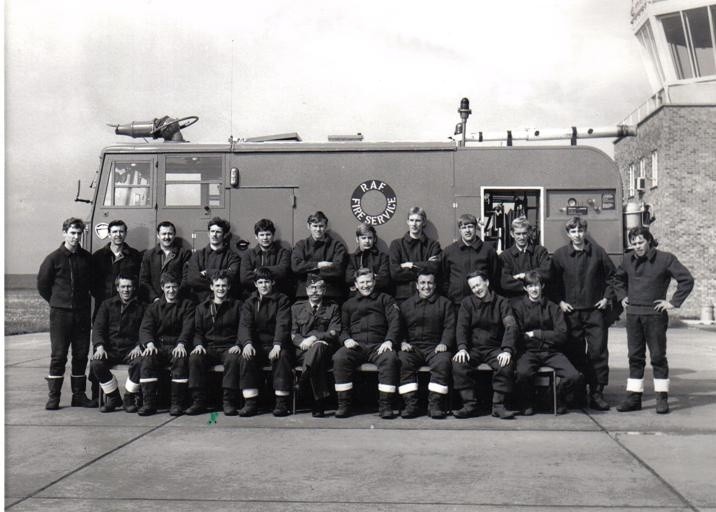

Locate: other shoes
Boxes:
[556,405,567,414]
[312,406,325,417]
[292,381,308,391]
[520,407,534,415]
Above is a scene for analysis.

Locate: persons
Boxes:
[613,226,694,414]
[37,218,99,410]
[91,207,617,419]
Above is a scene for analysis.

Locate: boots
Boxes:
[44,375,64,410]
[185,388,207,415]
[654,379,669,413]
[223,388,236,416]
[138,377,158,416]
[123,376,140,413]
[239,388,259,416]
[427,382,448,419]
[378,383,396,418]
[492,391,514,418]
[617,379,643,412]
[169,379,189,416]
[334,382,353,417]
[274,390,291,416]
[590,384,610,411]
[398,382,421,418]
[453,387,479,419]
[70,375,97,407]
[99,375,122,412]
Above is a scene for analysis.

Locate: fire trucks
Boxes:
[70,99,658,270]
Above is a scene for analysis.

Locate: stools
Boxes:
[91,355,566,420]
